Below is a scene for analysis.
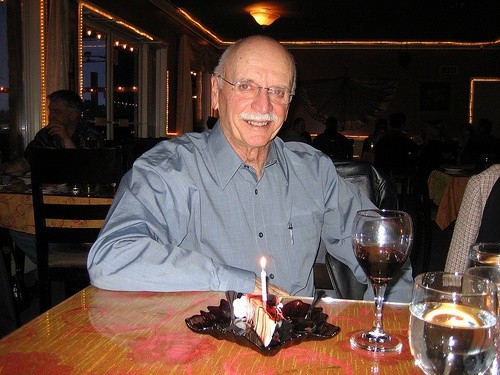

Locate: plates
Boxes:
[185,290,341,356]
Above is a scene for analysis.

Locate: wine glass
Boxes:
[350,210,412,354]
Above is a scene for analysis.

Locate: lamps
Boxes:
[250,7,280,30]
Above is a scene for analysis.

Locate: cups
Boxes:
[408,243,500,375]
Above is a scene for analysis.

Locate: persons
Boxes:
[86,35,415,305]
[472,177,500,255]
[3,87,107,285]
[282,102,490,190]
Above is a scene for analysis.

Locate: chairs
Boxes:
[13,145,127,312]
[325,160,399,301]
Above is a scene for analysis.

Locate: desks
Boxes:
[0,174,116,291]
[0,285,425,375]
[426,166,476,230]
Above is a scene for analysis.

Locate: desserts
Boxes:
[233,293,286,347]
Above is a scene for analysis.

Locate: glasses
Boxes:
[218,75,295,105]
